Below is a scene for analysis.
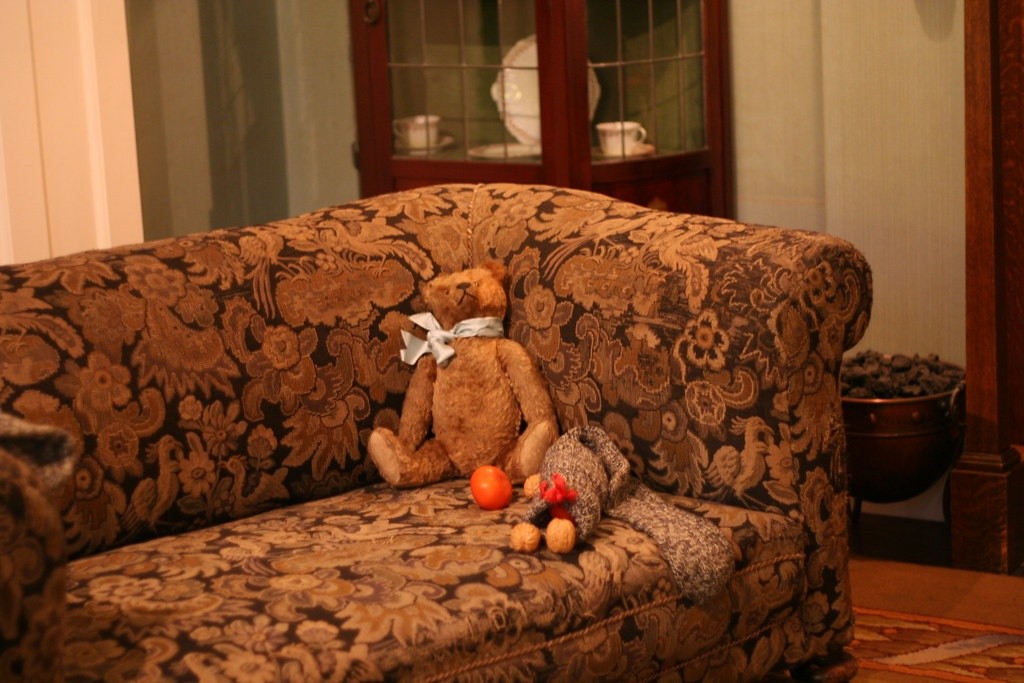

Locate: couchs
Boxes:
[0,182,873,683]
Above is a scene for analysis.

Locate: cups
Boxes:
[392,116,441,146]
[596,122,646,155]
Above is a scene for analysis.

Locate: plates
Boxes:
[491,35,601,146]
[592,145,655,159]
[468,143,540,158]
[393,133,454,155]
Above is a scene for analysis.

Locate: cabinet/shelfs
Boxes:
[350,0,728,218]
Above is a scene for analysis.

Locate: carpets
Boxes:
[850,608,1024,683]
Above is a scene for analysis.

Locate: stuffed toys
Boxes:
[366,259,560,487]
[511,425,735,604]
